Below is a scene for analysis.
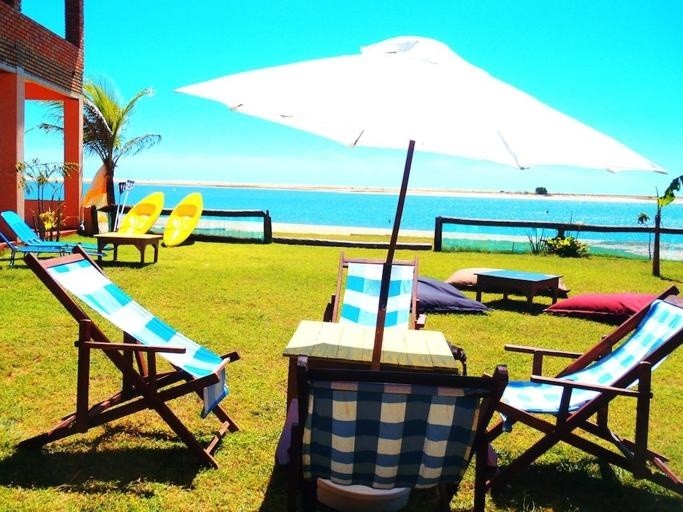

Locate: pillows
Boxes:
[542,294,661,323]
[443,267,572,295]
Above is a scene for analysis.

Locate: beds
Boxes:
[410,275,494,316]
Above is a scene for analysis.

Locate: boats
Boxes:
[163,193,205,246]
[118,191,164,234]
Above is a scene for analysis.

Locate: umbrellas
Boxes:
[171,35,673,372]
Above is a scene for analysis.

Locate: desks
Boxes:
[93,232,163,265]
[474,270,564,309]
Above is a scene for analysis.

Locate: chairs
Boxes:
[0,210,114,268]
[323,253,427,330]
[274,364,509,512]
[11,244,242,471]
[487,283,682,494]
